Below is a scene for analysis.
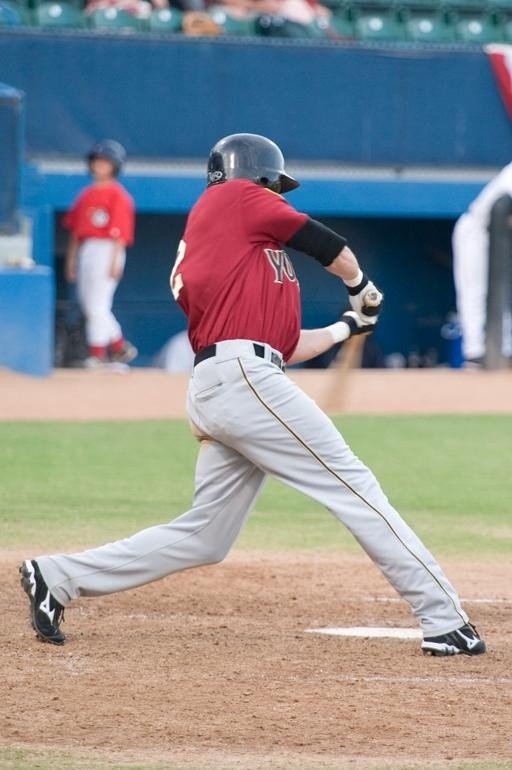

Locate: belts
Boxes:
[194,343,287,374]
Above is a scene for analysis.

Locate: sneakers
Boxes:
[17,558,66,646]
[420,620,487,657]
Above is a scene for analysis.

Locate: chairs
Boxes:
[10,0,510,51]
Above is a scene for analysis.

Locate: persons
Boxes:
[16,133,488,659]
[63,136,139,367]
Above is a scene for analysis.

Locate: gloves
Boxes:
[329,266,385,346]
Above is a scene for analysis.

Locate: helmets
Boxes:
[204,129,301,196]
[82,139,128,177]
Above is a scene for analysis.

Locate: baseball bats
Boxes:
[312,290,381,415]
